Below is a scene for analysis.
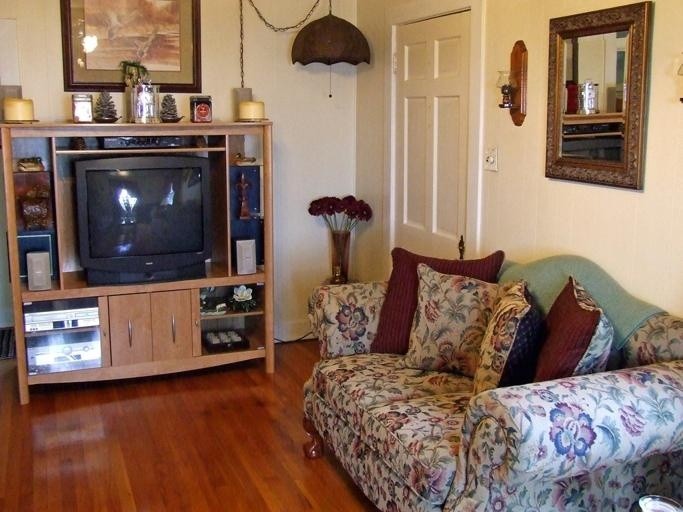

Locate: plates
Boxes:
[638,495,683,512]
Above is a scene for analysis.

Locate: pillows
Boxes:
[370,245,615,396]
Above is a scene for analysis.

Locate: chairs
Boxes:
[298,253,683,510]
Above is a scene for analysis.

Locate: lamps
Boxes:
[494,38,529,127]
[234,0,372,97]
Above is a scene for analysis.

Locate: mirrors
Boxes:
[545,0,650,191]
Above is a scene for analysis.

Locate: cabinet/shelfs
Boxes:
[561,109,625,162]
[2,119,276,406]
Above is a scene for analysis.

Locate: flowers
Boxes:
[307,192,374,267]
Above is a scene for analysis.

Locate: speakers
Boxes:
[236,239,256,275]
[27,251,51,291]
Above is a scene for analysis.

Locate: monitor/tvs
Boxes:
[75,154,212,284]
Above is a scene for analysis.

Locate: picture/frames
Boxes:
[60,0,202,93]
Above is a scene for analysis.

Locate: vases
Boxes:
[330,228,350,283]
